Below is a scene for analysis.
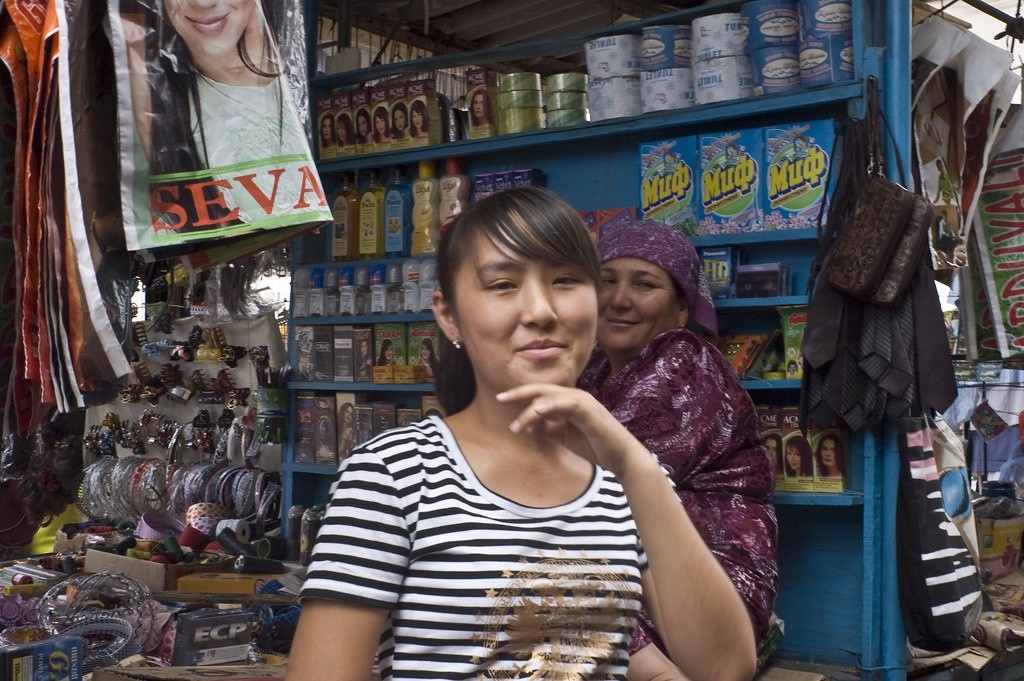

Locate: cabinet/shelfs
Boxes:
[279,0,926,681]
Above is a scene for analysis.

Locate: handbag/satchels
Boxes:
[900,416,982,652]
[814,76,932,307]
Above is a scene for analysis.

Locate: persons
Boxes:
[356,108,374,144]
[374,106,391,143]
[410,100,429,138]
[26,408,85,553]
[336,113,356,146]
[933,159,954,205]
[418,338,441,377]
[939,211,955,248]
[814,435,841,476]
[338,402,355,455]
[391,102,409,139]
[785,435,813,476]
[578,220,777,681]
[321,114,336,147]
[470,90,492,126]
[285,186,756,681]
[762,433,782,470]
[378,339,397,364]
[147,0,325,230]
[788,361,797,376]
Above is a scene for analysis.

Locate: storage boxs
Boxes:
[0,636,85,681]
[84,548,237,592]
[172,608,254,665]
[52,518,133,554]
[177,572,286,595]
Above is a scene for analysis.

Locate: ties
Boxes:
[800,105,960,434]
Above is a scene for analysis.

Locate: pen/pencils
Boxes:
[252,364,295,444]
